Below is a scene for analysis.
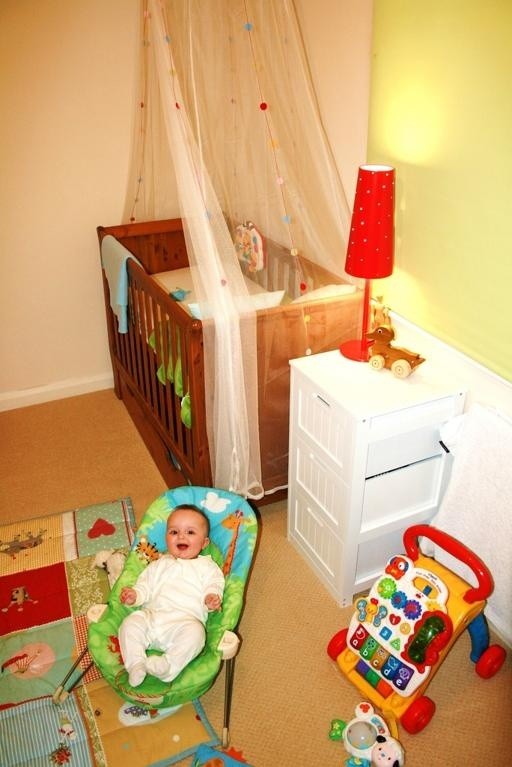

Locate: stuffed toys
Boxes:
[149,319,193,429]
[372,735,404,767]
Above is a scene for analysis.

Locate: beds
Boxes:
[91,210,367,509]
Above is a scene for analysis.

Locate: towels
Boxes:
[98,232,145,336]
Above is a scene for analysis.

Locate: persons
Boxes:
[117,504,225,688]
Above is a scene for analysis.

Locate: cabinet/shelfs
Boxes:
[287,349,462,607]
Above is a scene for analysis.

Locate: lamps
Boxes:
[340,161,395,360]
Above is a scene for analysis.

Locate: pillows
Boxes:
[184,283,355,318]
